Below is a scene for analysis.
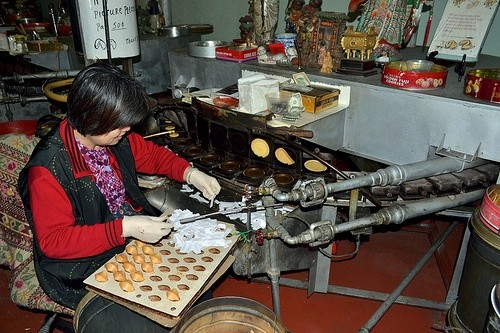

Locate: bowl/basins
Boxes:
[275,33,295,52]
[265,92,293,111]
[406,60,434,72]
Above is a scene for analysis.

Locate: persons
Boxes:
[17,63,220,310]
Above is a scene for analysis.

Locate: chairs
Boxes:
[0,133,75,333]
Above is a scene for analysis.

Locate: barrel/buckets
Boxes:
[172,296,288,333]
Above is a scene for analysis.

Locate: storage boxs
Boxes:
[381,59,449,90]
[464,68,500,103]
[277,82,340,112]
[215,45,256,63]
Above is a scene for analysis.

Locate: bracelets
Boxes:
[186,167,197,184]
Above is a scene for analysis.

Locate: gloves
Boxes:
[122,216,173,243]
[190,170,220,200]
